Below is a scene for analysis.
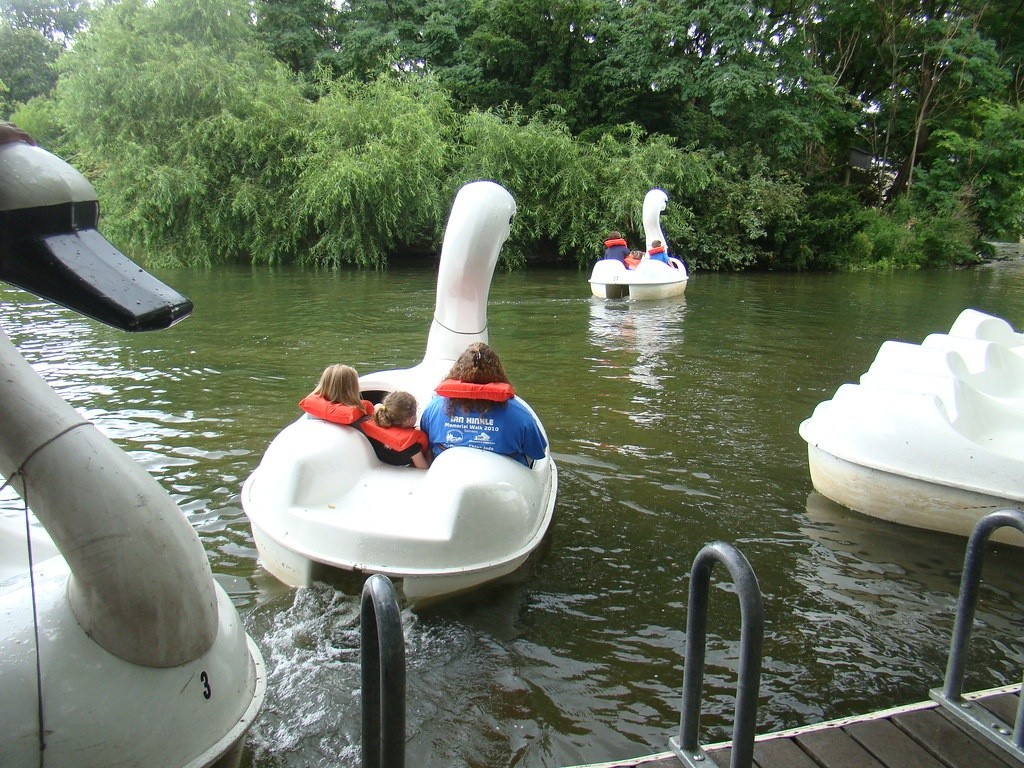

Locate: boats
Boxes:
[0,121,272,768]
[239,180,559,609]
[797,308,1024,550]
[587,189,689,301]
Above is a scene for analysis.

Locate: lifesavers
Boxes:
[436,377,514,401]
[360,417,430,453]
[648,245,666,256]
[624,255,641,266]
[298,392,374,424]
[604,239,627,248]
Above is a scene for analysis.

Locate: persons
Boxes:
[299,364,374,434]
[362,391,430,470]
[648,240,672,267]
[624,251,645,270]
[604,231,630,262]
[423,344,546,467]
